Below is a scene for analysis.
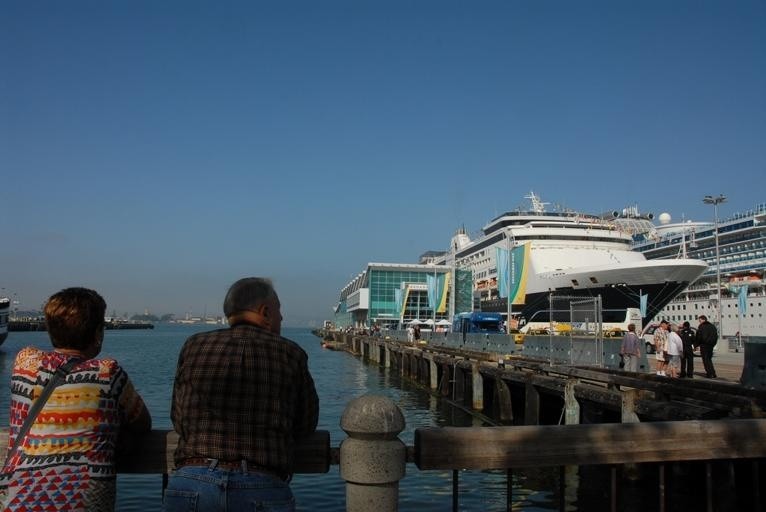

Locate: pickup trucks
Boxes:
[596,327,625,340]
[510,327,552,344]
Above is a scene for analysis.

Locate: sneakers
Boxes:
[657,371,665,376]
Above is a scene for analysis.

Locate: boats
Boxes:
[169,302,228,325]
[105,316,155,330]
[0,295,11,349]
[8,292,48,332]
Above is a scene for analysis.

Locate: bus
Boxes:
[515,307,644,337]
[499,312,526,326]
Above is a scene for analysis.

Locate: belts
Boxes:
[176,458,263,474]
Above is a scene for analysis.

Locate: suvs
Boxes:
[640,322,705,358]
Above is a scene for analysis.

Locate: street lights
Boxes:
[699,191,729,351]
[496,225,520,335]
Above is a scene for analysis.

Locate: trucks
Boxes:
[451,311,503,338]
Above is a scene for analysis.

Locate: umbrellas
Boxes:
[436,319,453,325]
[408,319,424,324]
[424,318,437,328]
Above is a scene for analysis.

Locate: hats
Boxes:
[661,320,669,324]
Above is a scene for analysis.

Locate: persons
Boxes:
[436,325,443,332]
[406,325,415,346]
[653,316,718,379]
[414,325,421,347]
[163,277,319,512]
[620,324,641,373]
[1,288,152,512]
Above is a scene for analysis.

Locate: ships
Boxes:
[414,185,713,329]
[629,202,766,352]
